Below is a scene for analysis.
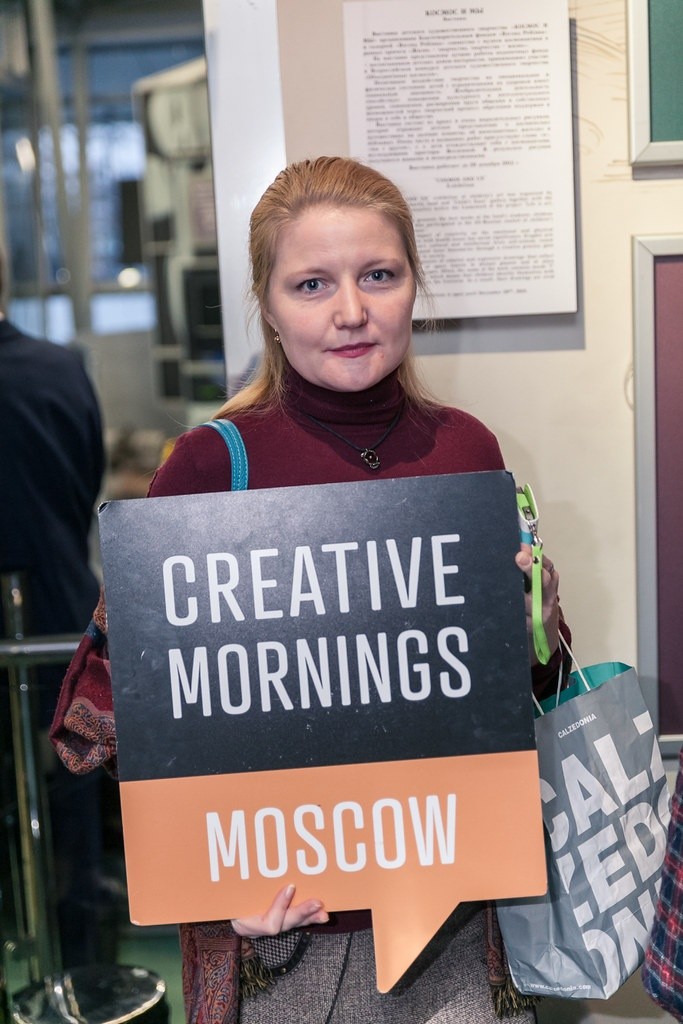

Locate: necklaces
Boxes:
[302,406,402,470]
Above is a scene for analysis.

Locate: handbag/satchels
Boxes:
[497,629,670,997]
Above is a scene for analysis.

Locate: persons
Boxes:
[49,158,571,1024]
[0,248,125,973]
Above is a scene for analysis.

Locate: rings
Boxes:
[549,565,554,575]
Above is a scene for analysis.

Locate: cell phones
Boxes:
[515,484,534,526]
